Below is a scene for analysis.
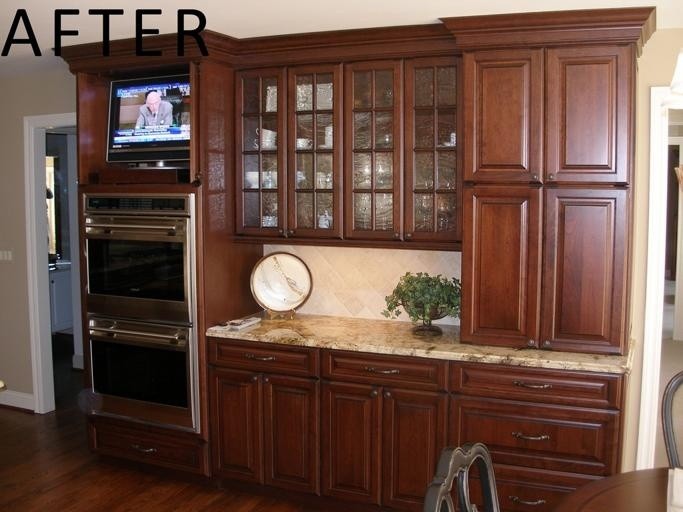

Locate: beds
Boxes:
[554,467,683,512]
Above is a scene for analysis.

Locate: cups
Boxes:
[253,123,311,152]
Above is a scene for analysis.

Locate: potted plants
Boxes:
[381,271,461,337]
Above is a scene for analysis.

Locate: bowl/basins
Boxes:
[244,172,266,188]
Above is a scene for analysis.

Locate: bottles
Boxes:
[317,171,327,189]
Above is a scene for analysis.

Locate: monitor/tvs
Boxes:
[105,74,191,170]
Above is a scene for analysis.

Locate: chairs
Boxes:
[424,442,500,512]
[661,371,683,467]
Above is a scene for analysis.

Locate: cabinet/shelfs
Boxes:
[344,55,463,243]
[463,41,636,184]
[461,184,632,357]
[234,62,343,241]
[448,361,623,512]
[207,337,320,497]
[321,347,449,512]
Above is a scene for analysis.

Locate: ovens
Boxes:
[79,188,194,422]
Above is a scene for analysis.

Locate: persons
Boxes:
[135,89,173,130]
